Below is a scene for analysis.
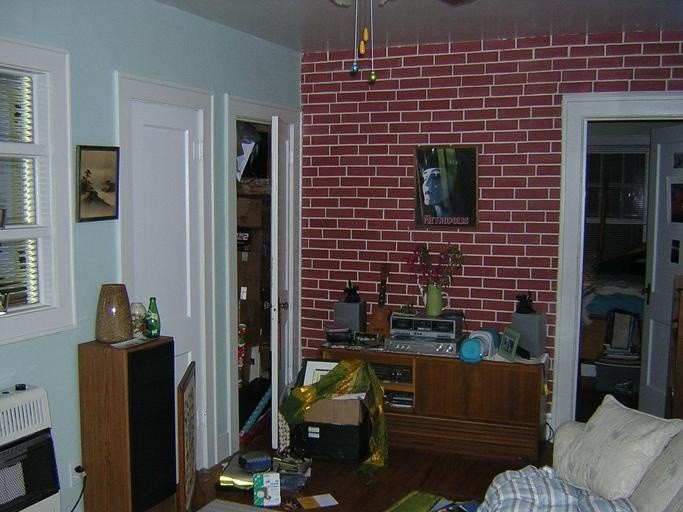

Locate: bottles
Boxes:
[144,296,162,337]
[128,301,148,339]
[94,283,133,344]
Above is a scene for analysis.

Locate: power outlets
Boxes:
[69,458,83,487]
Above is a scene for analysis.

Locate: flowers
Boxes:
[405,243,463,284]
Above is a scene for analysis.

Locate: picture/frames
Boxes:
[301,359,339,387]
[177,361,196,512]
[413,143,479,229]
[498,328,521,361]
[76,145,120,222]
[666,176,683,228]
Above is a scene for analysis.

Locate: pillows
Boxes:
[553,393,683,501]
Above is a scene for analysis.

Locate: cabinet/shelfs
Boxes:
[319,341,551,464]
[76,335,177,512]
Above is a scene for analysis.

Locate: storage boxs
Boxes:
[594,359,641,394]
[304,420,368,463]
[305,398,366,426]
[237,191,270,389]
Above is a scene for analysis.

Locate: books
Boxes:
[323,324,348,333]
[390,396,413,408]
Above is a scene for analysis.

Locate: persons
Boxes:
[421,150,475,227]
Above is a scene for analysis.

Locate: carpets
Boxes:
[196,498,282,512]
[379,490,481,512]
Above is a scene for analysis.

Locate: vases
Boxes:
[95,283,160,344]
[425,284,443,317]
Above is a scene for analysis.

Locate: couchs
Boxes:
[474,422,683,512]
